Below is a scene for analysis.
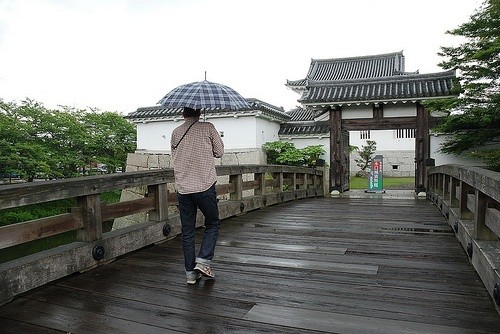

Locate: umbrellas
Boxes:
[156,71,254,123]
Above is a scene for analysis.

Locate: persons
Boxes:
[170,84,224,285]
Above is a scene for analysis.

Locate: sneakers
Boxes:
[193,262,215,278]
[185,267,202,284]
